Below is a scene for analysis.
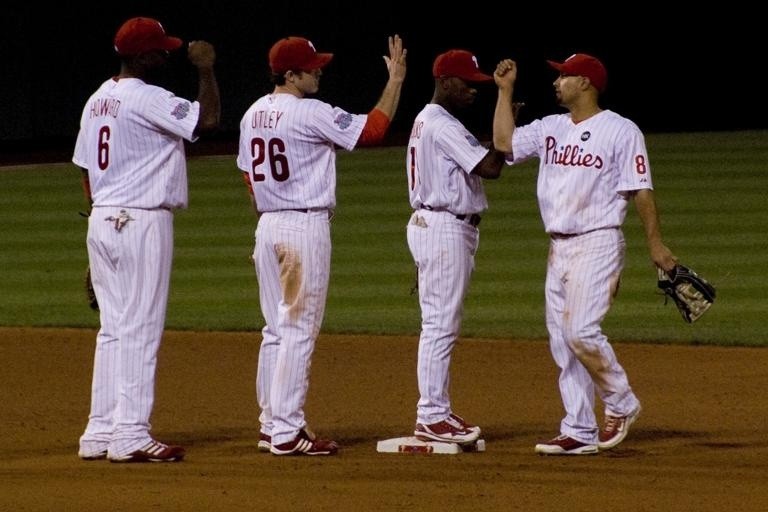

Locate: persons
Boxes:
[235,32,409,456]
[404,48,526,445]
[492,52,680,456]
[71,17,222,462]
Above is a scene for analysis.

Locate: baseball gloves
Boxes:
[657,262,714,323]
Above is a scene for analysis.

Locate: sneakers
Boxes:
[597,403,643,450]
[107,437,184,461]
[534,433,598,455]
[415,410,482,446]
[258,427,337,455]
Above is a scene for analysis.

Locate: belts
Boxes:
[552,231,573,240]
[458,212,482,227]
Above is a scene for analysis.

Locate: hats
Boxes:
[269,37,333,70]
[432,50,493,82]
[113,18,182,56]
[547,53,608,90]
